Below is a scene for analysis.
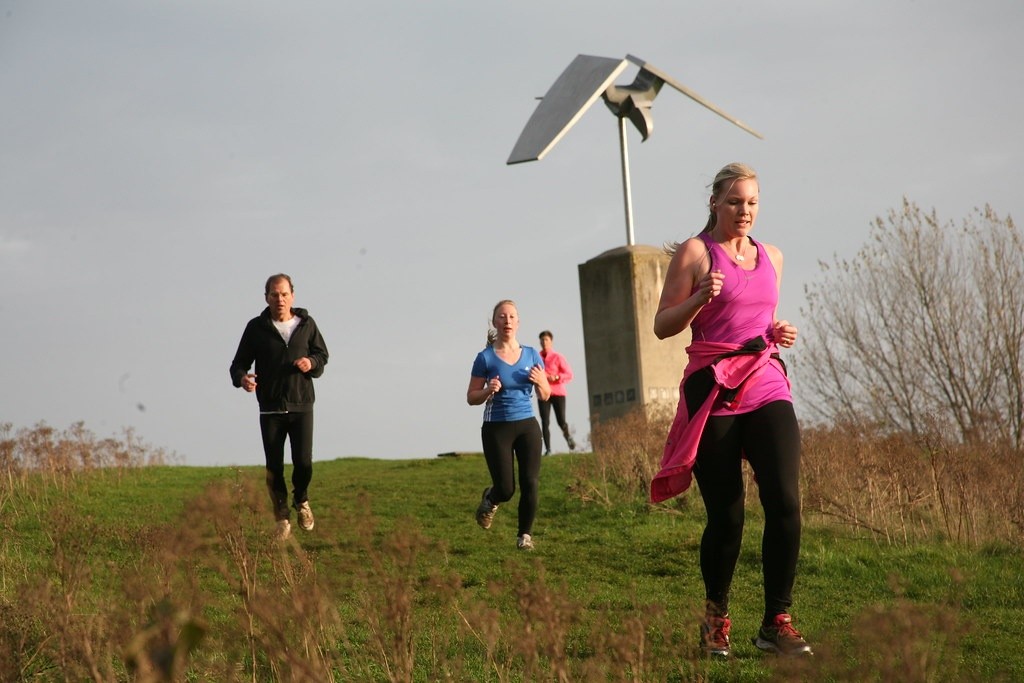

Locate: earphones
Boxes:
[713,203,718,208]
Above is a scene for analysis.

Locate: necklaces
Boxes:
[719,235,747,261]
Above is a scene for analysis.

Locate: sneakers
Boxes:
[700,608,730,656]
[292,497,314,532]
[755,615,813,658]
[274,519,290,542]
[475,488,498,529]
[517,535,535,552]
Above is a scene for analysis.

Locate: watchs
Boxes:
[555,375,559,379]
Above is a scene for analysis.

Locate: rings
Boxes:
[785,338,790,344]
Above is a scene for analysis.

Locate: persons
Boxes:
[653,164,813,659]
[229,273,329,540]
[538,331,575,457]
[467,300,552,552]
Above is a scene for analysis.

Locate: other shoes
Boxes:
[566,433,576,451]
[545,448,551,457]
